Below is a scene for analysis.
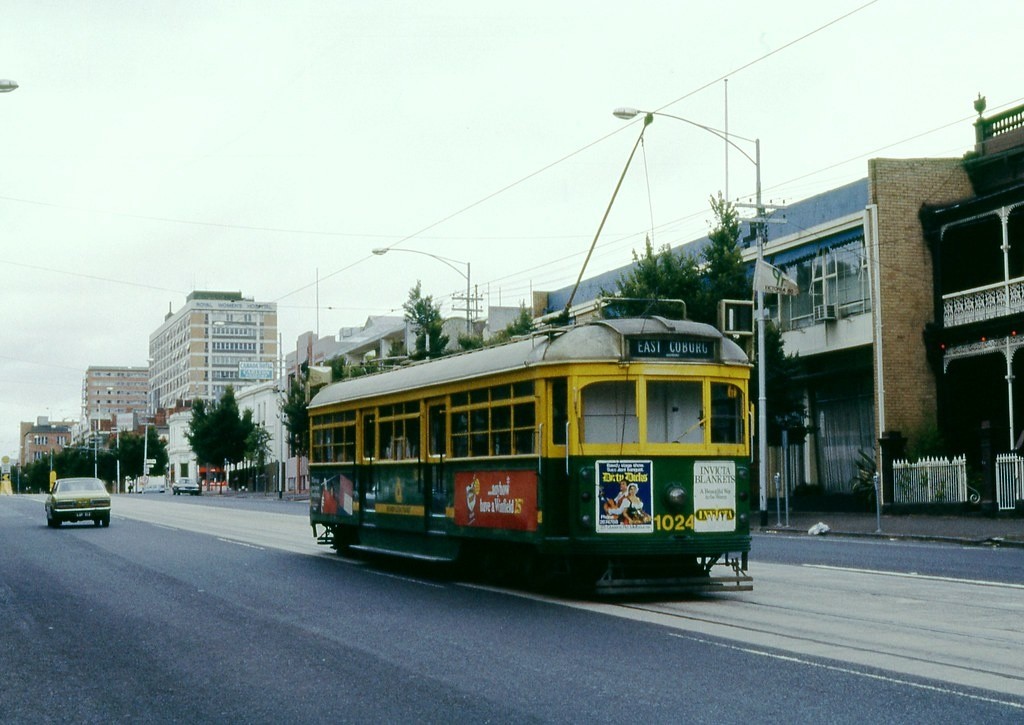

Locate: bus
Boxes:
[307,314,755,598]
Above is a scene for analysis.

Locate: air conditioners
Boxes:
[813,304,834,320]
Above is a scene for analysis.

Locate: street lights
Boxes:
[372,247,470,336]
[614,107,769,528]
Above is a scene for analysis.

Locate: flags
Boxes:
[754,260,799,296]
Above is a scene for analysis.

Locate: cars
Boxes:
[45,477,111,528]
[172,477,199,495]
